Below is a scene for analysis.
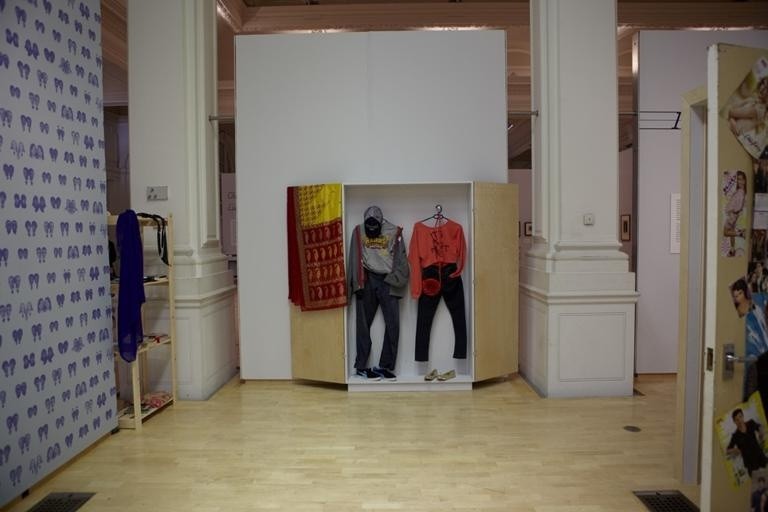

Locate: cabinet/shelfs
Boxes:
[106,211,180,432]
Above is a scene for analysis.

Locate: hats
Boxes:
[362,204,384,239]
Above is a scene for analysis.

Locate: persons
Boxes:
[760,489,768,512]
[723,169,747,257]
[726,408,768,478]
[752,476,767,512]
[757,162,764,185]
[748,272,757,293]
[748,262,768,293]
[732,279,756,318]
[728,75,768,136]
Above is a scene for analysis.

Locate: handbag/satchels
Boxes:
[422,279,440,296]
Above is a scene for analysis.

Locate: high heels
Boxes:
[727,228,746,257]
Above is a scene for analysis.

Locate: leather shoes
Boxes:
[424,368,457,381]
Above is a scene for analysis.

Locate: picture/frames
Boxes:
[524,222,532,235]
[621,214,630,241]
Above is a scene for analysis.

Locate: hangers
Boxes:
[419,203,449,227]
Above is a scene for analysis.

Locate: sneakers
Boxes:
[357,366,397,381]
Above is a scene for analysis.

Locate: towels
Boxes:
[116,211,146,365]
